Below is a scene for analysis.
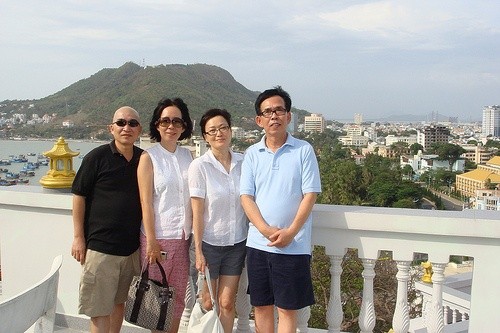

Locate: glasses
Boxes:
[205,126,231,136]
[112,118,139,127]
[259,108,288,118]
[156,116,184,128]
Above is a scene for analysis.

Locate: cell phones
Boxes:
[160,251,167,261]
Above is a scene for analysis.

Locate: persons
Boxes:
[138,98,194,333]
[240,87,323,333]
[187,109,249,333]
[71,106,145,333]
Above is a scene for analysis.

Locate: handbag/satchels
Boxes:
[187,266,225,333]
[124,258,175,332]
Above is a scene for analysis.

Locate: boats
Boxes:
[0,151,51,186]
